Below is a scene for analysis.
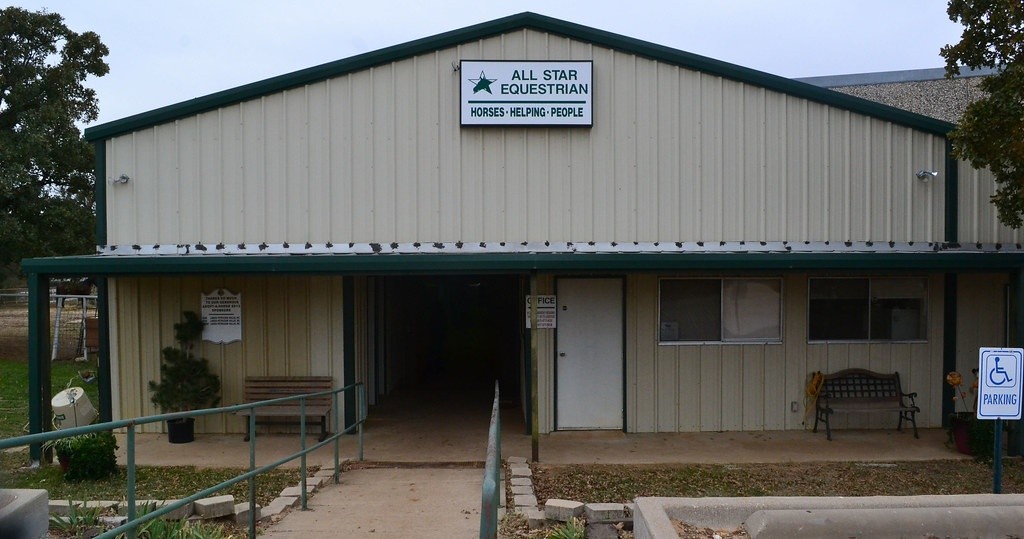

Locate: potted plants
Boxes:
[944,372,996,464]
[148,309,222,444]
[42,433,119,482]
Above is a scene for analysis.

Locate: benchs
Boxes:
[232,376,333,443]
[812,368,920,441]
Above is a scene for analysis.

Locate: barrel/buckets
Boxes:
[51,387,97,429]
